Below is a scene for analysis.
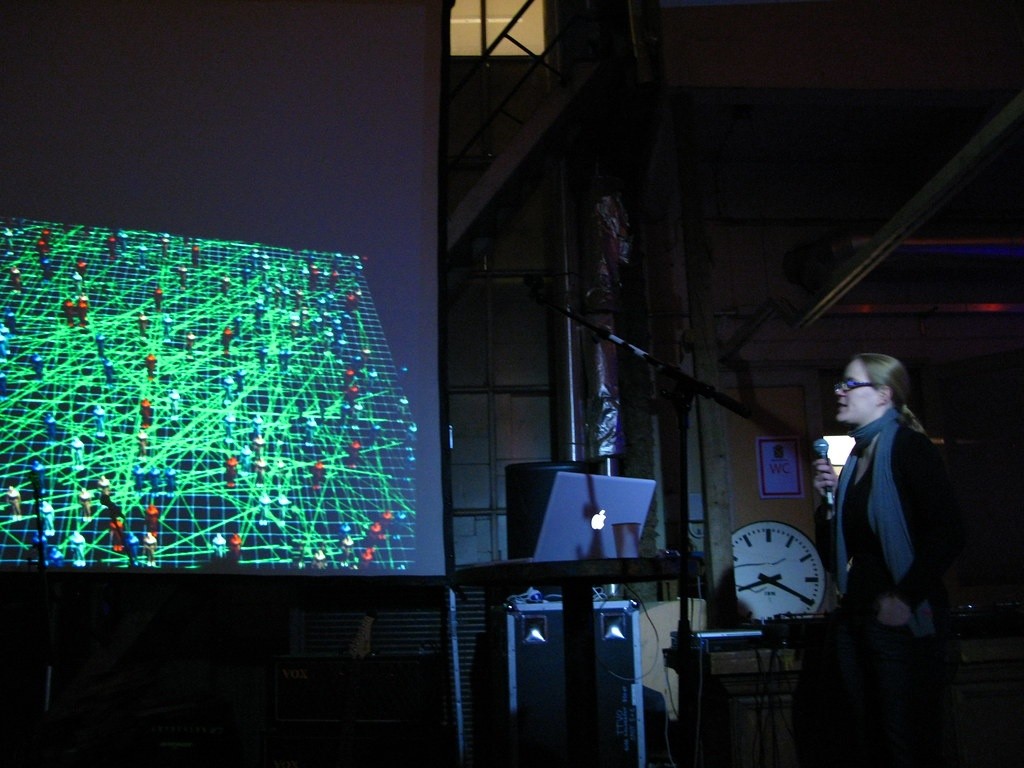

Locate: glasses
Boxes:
[832,376,874,394]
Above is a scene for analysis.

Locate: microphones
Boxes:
[814,440,833,506]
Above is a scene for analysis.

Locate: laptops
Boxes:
[454,472,657,571]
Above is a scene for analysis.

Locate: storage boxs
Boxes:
[507,600,645,768]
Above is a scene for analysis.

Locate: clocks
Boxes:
[699,521,826,619]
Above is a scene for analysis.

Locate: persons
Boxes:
[809,353,955,768]
